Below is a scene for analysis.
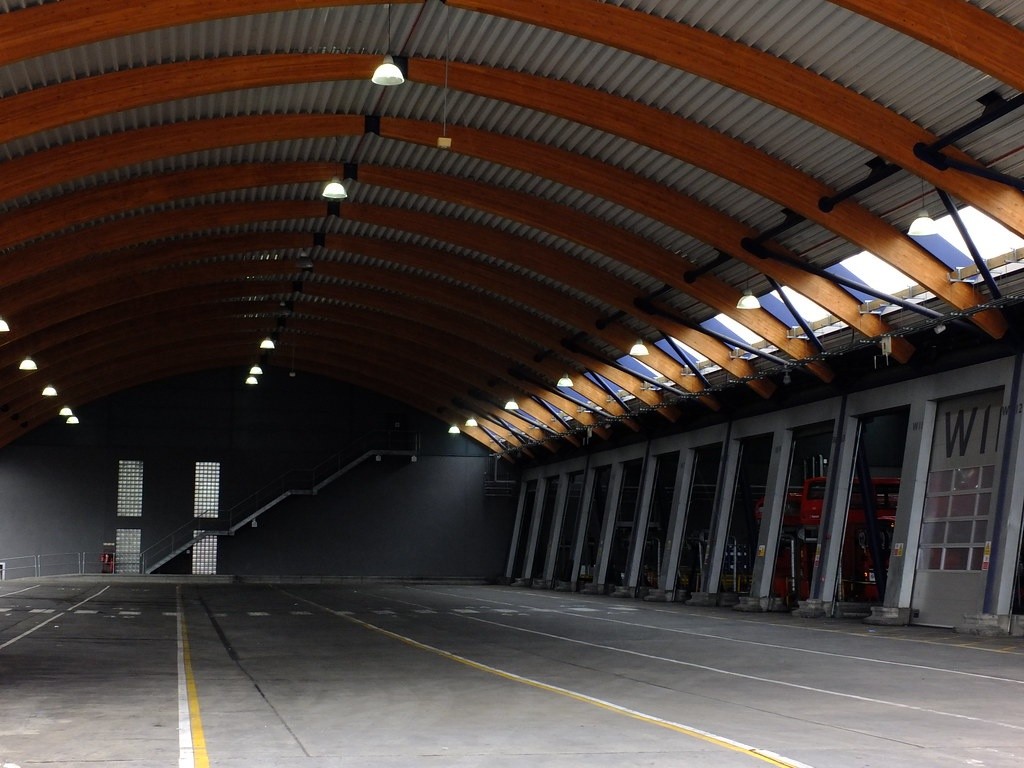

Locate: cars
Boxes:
[756,493,803,545]
[800,476,900,554]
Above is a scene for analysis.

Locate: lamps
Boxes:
[321,136,348,199]
[295,251,314,267]
[0,315,11,332]
[245,374,258,384]
[371,4,405,85]
[555,372,574,386]
[249,363,263,374]
[629,318,650,355]
[736,264,761,309]
[19,355,37,369]
[65,414,79,423]
[906,179,938,236]
[448,424,461,433]
[59,405,73,415]
[260,336,275,349]
[464,417,478,427]
[41,384,58,396]
[504,398,520,410]
